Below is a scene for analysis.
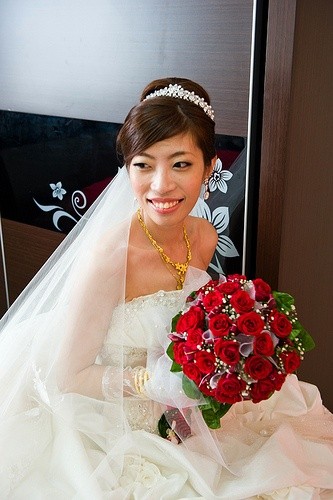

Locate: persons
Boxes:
[0,75,333,500]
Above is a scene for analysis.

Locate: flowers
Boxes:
[156,273,316,445]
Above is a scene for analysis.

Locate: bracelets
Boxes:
[138,367,145,394]
[134,369,141,397]
[143,369,149,384]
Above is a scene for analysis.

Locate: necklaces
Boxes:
[136,208,192,291]
